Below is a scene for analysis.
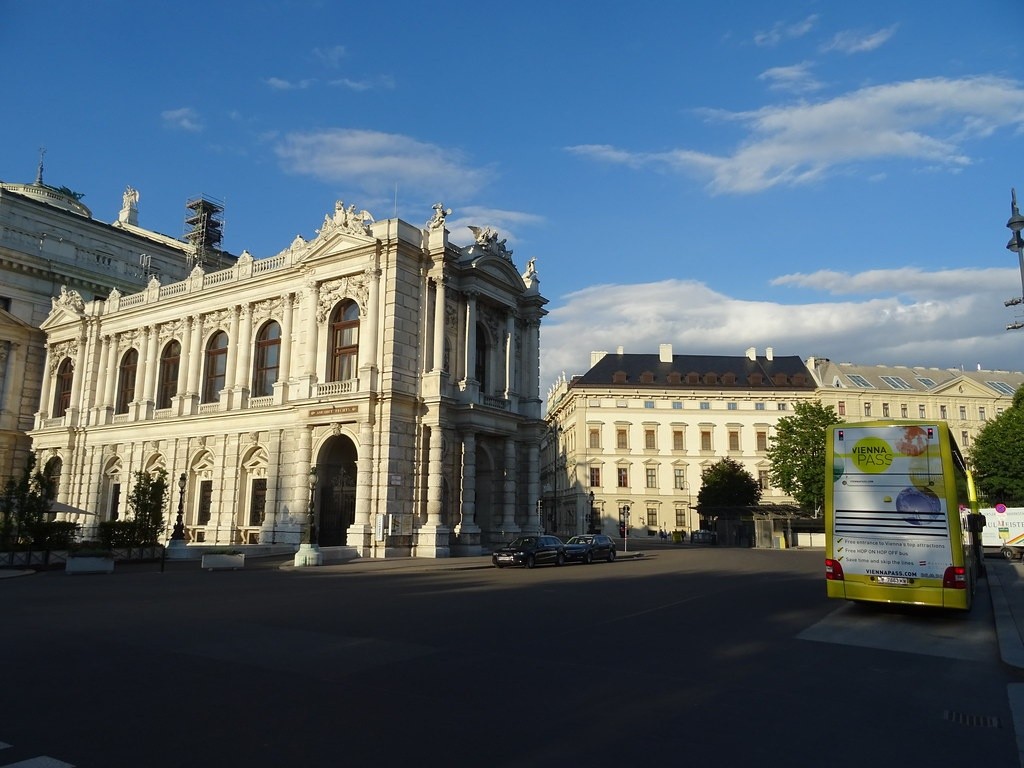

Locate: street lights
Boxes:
[588,490,597,534]
[170,472,187,540]
[301,467,319,545]
[680,481,693,533]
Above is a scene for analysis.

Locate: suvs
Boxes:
[492,534,567,569]
[563,534,617,564]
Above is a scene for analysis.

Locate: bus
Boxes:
[825,419,986,611]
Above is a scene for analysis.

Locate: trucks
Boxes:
[978,507,1024,561]
